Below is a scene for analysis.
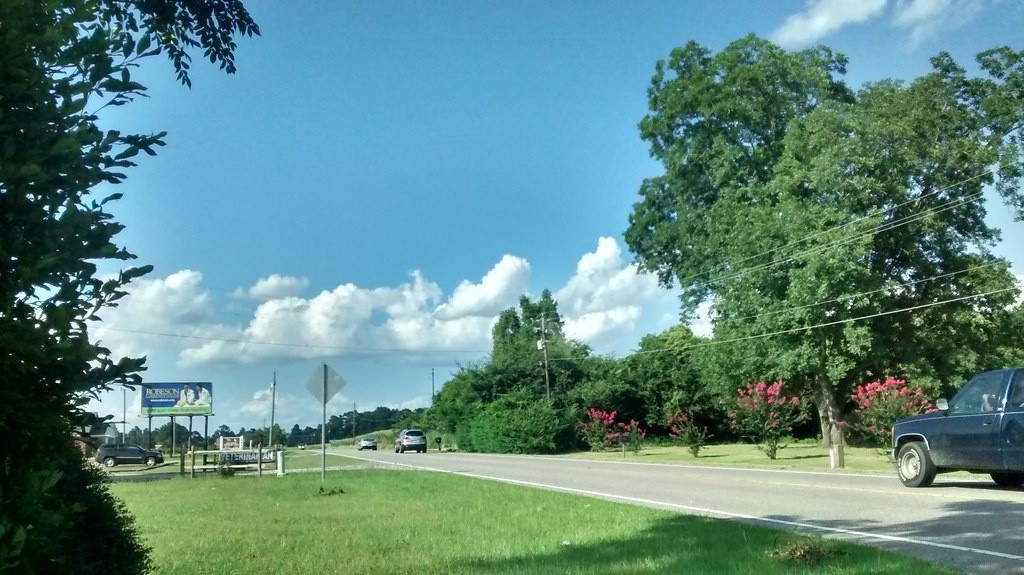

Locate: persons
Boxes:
[177,384,194,405]
[194,384,211,405]
[982,394,993,411]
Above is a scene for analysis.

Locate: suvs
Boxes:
[94,443,164,468]
[394,428,428,454]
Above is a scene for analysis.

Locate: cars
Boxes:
[358,438,377,451]
[886,367,1024,488]
